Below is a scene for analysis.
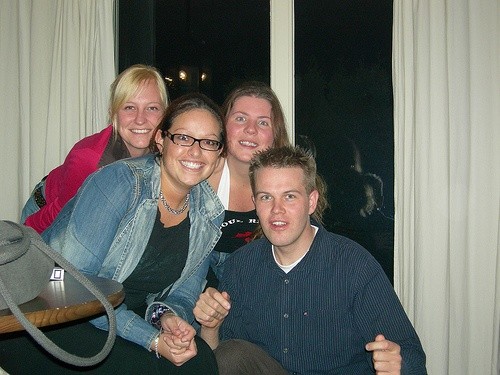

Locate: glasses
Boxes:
[162,129,223,152]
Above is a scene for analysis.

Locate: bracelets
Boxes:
[155,337,161,359]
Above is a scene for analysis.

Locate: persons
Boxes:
[20,64,426,375]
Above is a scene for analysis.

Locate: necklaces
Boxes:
[159,189,190,215]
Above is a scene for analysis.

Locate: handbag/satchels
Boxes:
[0,219,117,366]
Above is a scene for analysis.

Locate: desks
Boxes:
[0,272,125,333]
